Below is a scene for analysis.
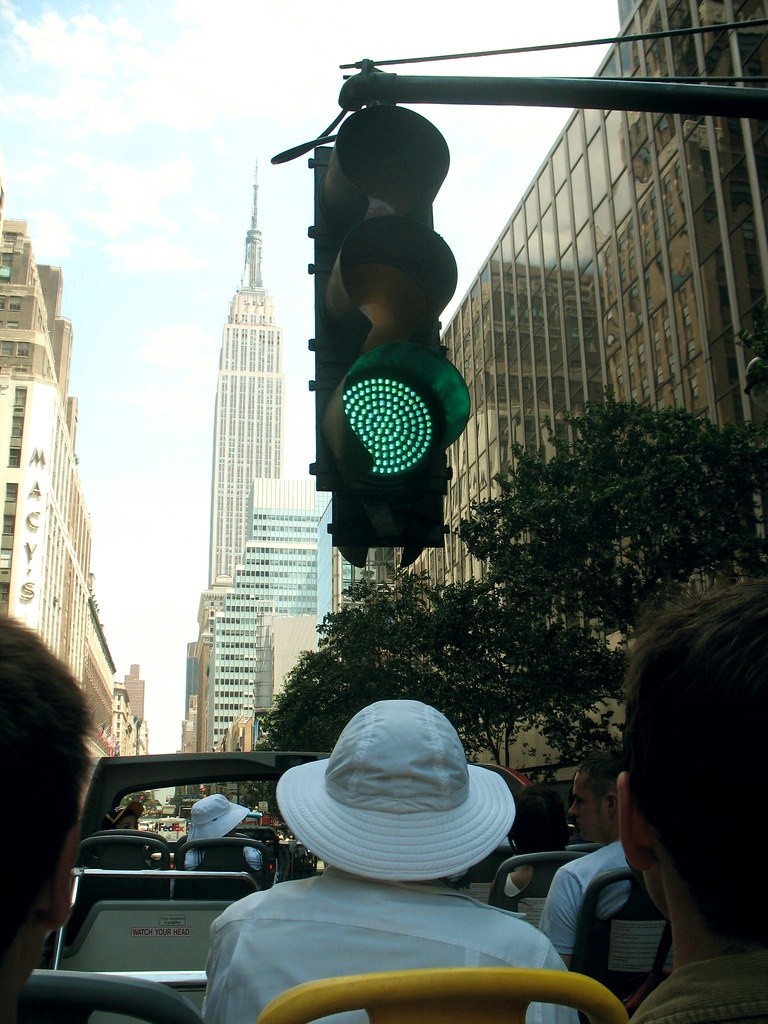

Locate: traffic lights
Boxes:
[303,103,472,570]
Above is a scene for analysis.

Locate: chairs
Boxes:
[16,830,675,1024]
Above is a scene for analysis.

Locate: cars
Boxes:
[137,805,262,840]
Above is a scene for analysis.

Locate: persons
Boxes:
[0,616,99,1024]
[610,580,768,1024]
[101,790,264,904]
[488,751,670,970]
[203,699,585,1024]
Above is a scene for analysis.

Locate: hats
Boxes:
[275,700,516,882]
[187,794,250,842]
[100,801,143,831]
